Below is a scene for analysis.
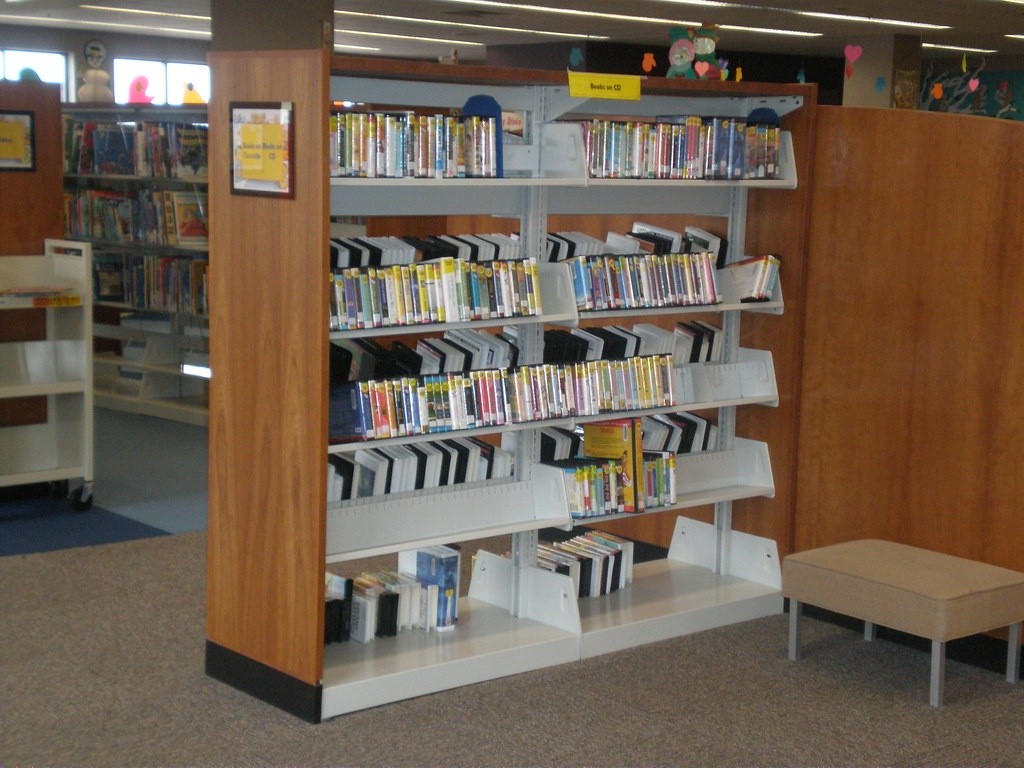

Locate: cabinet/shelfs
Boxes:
[202,0,820,724]
[0,77,208,511]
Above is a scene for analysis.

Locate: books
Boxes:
[119,312,174,336]
[60,115,210,319]
[324,103,785,650]
[183,319,210,338]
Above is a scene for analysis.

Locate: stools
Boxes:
[778,536,1024,710]
